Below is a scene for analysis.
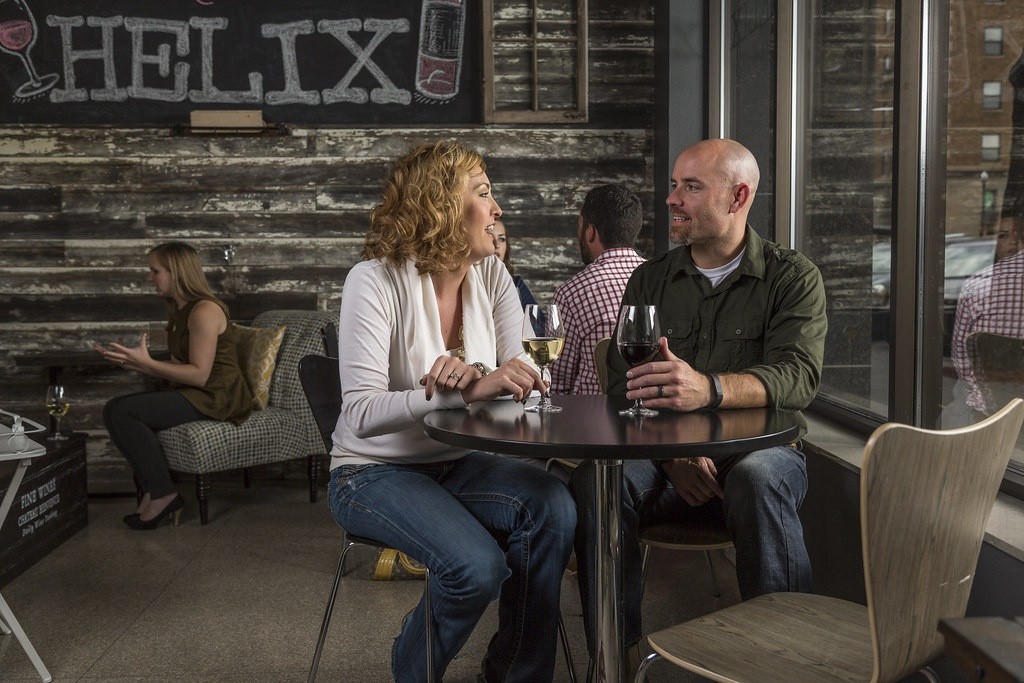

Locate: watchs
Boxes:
[473,362,492,376]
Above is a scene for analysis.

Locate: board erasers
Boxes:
[189,109,263,134]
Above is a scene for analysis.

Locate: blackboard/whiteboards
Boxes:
[1,0,488,130]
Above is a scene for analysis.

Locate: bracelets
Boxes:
[708,374,723,409]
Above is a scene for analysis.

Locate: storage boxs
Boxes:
[190,109,263,133]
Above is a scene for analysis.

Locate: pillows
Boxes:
[232,322,286,410]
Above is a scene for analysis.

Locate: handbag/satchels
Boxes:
[369,547,424,581]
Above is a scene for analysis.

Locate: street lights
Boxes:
[979,171,989,236]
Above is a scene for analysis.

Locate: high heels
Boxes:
[123,494,184,531]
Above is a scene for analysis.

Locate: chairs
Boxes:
[158,309,1024,683]
[965,331,1024,473]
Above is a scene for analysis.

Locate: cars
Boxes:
[824,243,890,339]
[943,239,997,323]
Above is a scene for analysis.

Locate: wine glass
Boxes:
[521,303,565,412]
[45,385,69,440]
[617,305,661,417]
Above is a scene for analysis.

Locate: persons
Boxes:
[492,218,546,338]
[94,242,253,530]
[544,184,649,395]
[568,138,828,683]
[326,140,577,683]
[951,197,1024,415]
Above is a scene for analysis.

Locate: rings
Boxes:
[451,373,460,381]
[658,386,663,396]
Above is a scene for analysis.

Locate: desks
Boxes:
[0,422,91,683]
[423,392,800,683]
